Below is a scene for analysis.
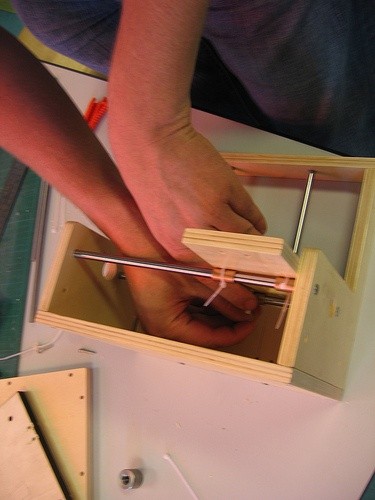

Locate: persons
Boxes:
[0,0,375,348]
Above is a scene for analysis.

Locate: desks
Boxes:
[17,61,375,500]
